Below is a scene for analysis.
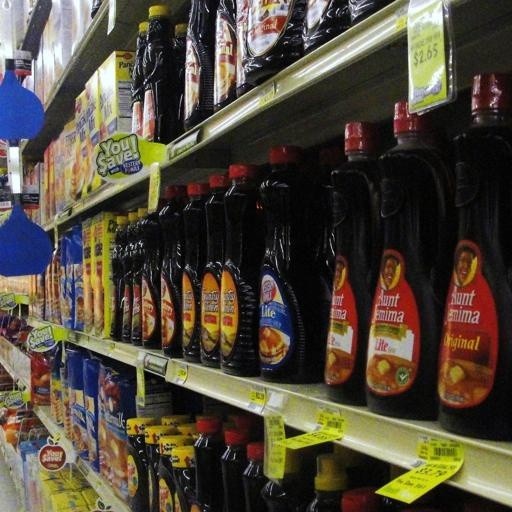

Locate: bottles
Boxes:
[125,413,435,512]
[110,162,257,379]
[324,123,381,406]
[128,0,391,144]
[436,73,512,442]
[258,146,323,386]
[363,99,438,423]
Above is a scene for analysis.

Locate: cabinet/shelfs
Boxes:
[0,1,512,511]
